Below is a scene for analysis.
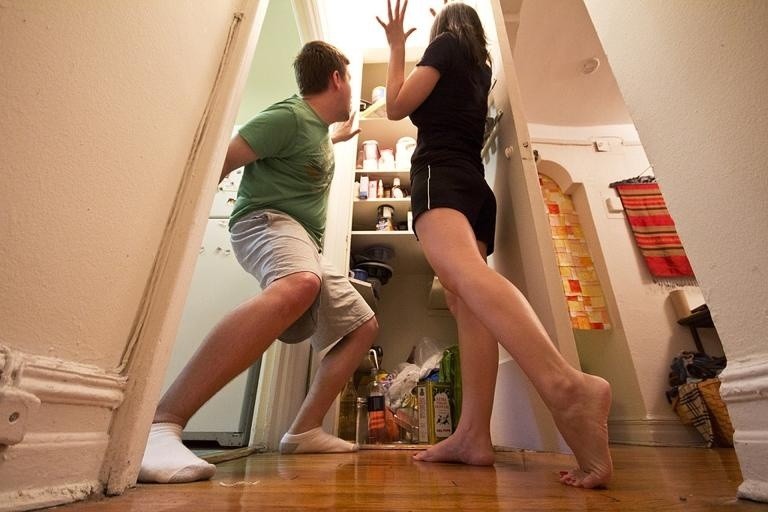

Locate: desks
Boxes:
[677,308,713,353]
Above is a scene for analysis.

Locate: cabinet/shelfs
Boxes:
[352,60,435,274]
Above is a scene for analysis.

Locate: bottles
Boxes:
[377,176,405,198]
[338,367,388,445]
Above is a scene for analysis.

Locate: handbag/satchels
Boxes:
[664,378,734,448]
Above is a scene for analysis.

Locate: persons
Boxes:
[376,0,612,489]
[137,40,379,483]
